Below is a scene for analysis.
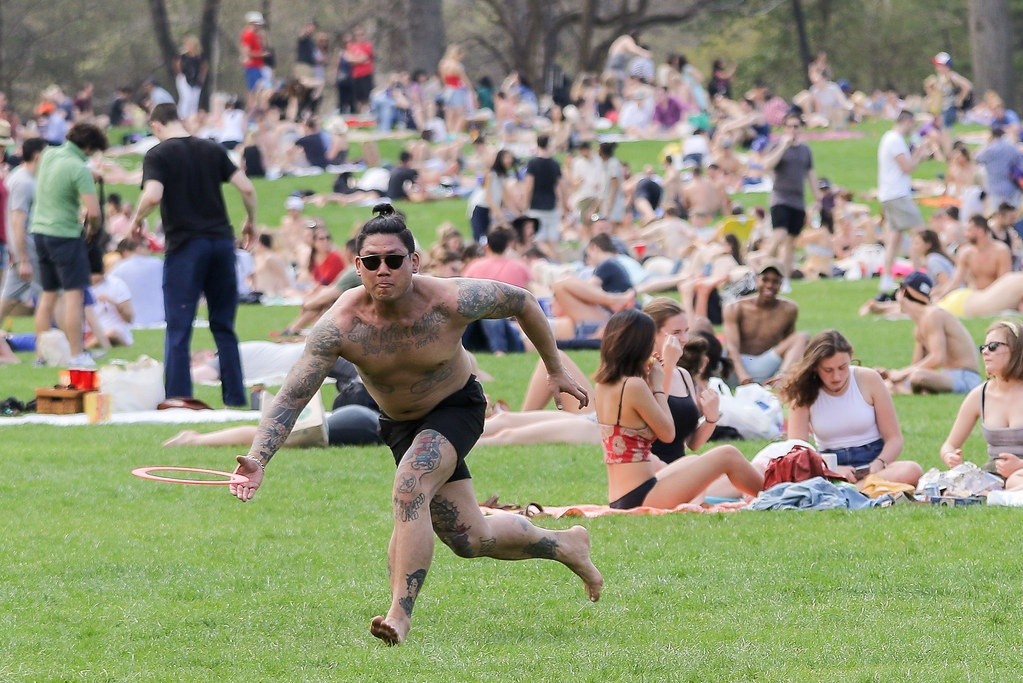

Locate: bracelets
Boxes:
[876,457,887,469]
[706,413,723,423]
[653,392,665,395]
[248,456,265,472]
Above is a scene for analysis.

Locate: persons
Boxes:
[773,329,924,489]
[940,321,1023,491]
[0,12,1023,391]
[590,298,765,510]
[125,103,257,409]
[230,204,603,645]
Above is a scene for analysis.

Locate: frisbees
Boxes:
[130,466,248,487]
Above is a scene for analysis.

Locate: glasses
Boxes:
[358,253,409,270]
[980,342,1008,353]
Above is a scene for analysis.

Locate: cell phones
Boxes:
[854,465,871,480]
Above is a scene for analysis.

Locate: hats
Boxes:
[903,272,932,303]
[758,262,784,277]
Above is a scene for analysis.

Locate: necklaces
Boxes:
[822,378,848,395]
